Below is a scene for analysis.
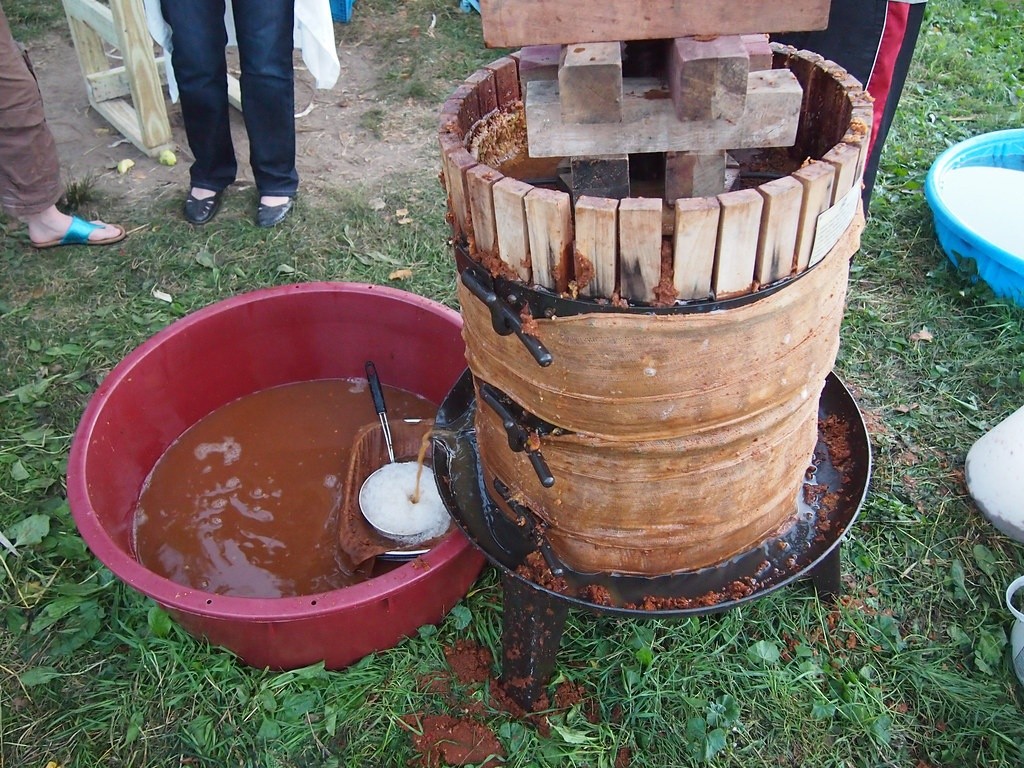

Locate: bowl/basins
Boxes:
[923,127,1024,311]
[66,282,488,672]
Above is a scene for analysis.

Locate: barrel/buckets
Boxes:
[1006,575,1024,687]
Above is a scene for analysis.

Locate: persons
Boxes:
[0,1,127,247]
[159,0,300,228]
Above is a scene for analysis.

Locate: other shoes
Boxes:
[257,195,293,228]
[184,188,221,225]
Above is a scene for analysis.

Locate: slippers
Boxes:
[31,216,125,248]
